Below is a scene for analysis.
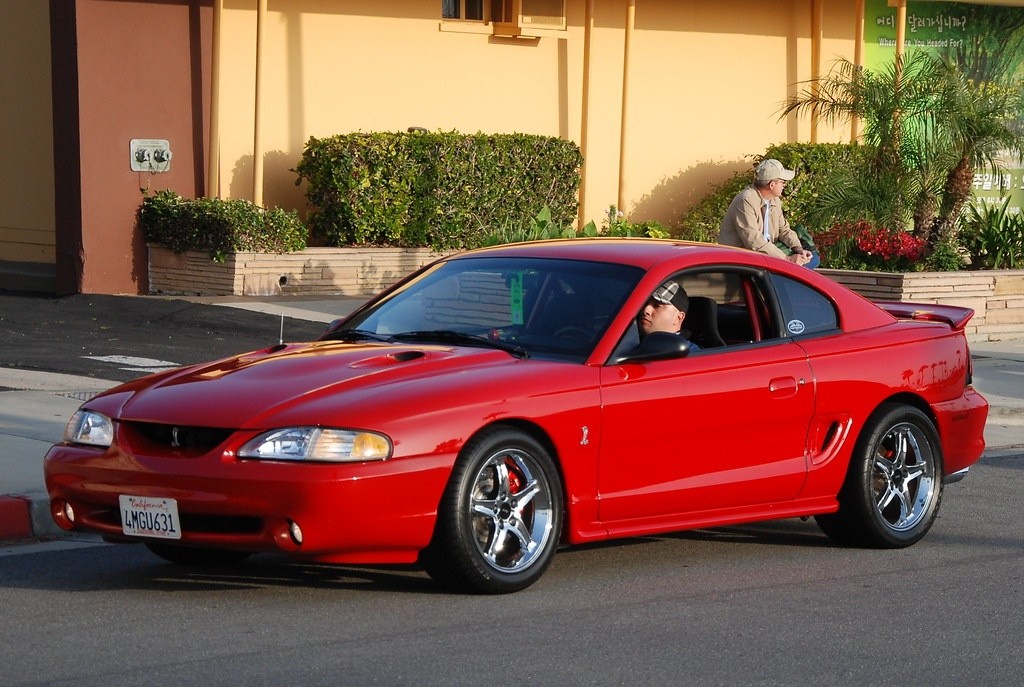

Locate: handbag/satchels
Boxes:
[772,224,817,256]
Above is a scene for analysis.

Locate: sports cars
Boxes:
[42,236,990,598]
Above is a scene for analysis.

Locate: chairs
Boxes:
[717,304,759,346]
[681,296,727,350]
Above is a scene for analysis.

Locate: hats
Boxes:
[652,281,690,316]
[755,159,795,181]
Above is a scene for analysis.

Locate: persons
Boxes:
[718,159,814,266]
[628,281,702,355]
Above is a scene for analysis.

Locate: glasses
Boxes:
[767,180,785,185]
